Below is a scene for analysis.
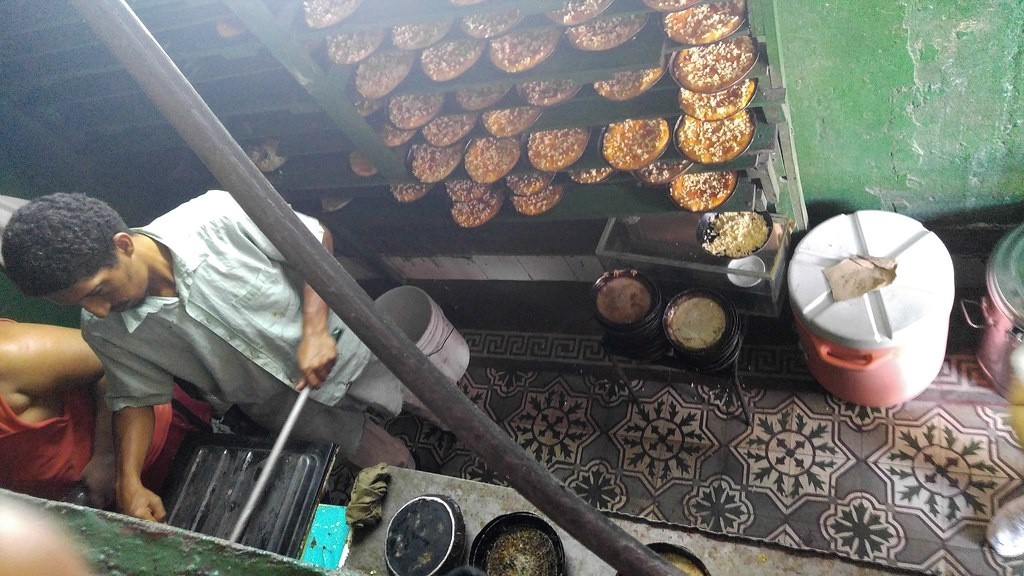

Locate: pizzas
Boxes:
[214,0,755,229]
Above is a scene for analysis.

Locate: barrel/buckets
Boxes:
[376,286,470,381]
[786,211,957,406]
[961,221,1024,406]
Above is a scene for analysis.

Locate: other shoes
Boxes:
[985,494,1024,556]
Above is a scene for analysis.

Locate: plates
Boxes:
[616,542,712,576]
[589,256,766,373]
[468,512,566,576]
[216,0,773,258]
[384,494,465,576]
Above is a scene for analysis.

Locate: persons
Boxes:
[0,190,453,525]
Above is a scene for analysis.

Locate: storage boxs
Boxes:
[597,210,792,317]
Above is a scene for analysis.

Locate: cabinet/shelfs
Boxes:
[0,0,792,252]
